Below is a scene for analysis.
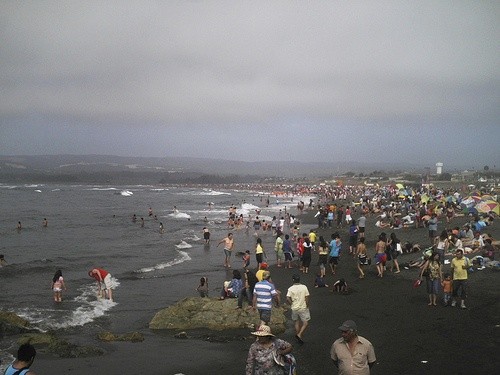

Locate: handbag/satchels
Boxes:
[426,266,432,280]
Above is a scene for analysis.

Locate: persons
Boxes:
[149,185,463,211]
[480,185,498,201]
[0,211,500,375]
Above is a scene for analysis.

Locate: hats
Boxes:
[339,320,356,332]
[251,325,276,338]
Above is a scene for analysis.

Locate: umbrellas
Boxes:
[390,183,404,191]
[457,191,500,216]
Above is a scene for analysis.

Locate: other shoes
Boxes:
[295,334,304,345]
